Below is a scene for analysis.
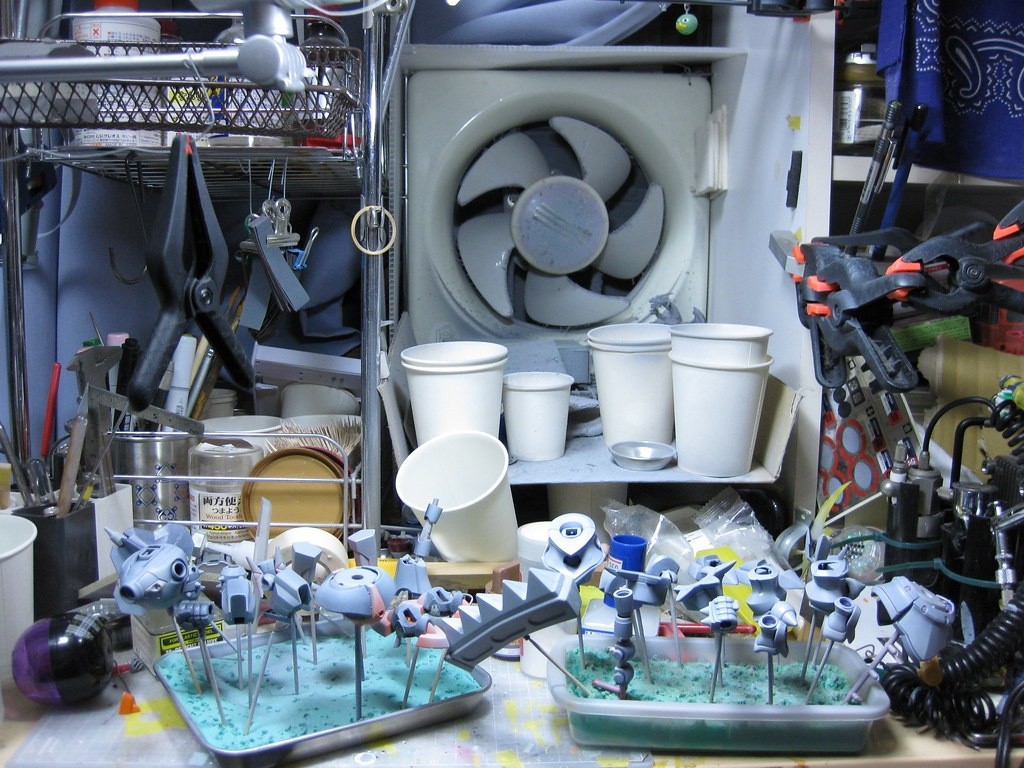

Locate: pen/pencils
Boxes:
[841,99,899,257]
[872,102,938,260]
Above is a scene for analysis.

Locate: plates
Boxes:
[609,439,677,472]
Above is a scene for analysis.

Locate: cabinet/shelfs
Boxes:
[0,34,385,555]
[713,0,1024,541]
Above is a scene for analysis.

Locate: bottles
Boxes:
[69,0,162,148]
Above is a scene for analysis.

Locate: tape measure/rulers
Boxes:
[88,385,206,441]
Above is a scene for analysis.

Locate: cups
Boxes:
[197,416,285,458]
[585,321,776,478]
[13,500,98,625]
[0,513,38,688]
[109,434,198,532]
[196,387,239,420]
[278,381,361,417]
[501,370,575,462]
[395,435,520,561]
[399,341,509,449]
